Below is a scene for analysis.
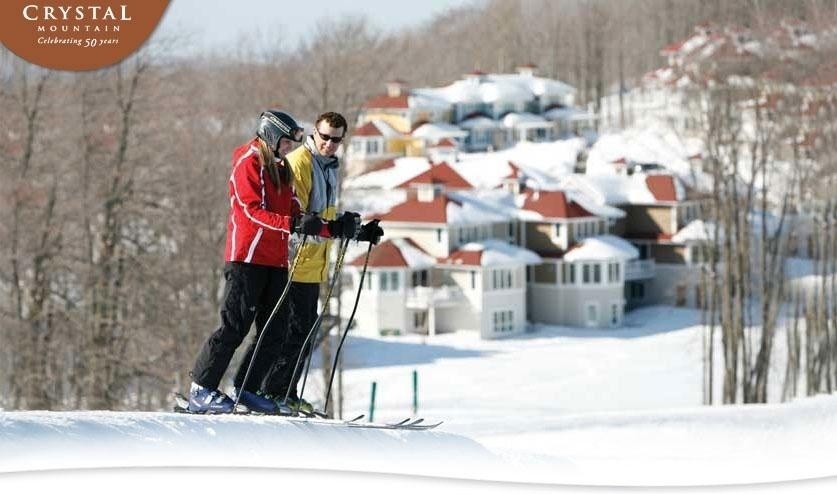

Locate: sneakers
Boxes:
[190,383,235,413]
[236,389,313,414]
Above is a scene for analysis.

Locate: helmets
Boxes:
[256,111,302,152]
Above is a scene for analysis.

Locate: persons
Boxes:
[188,109,362,411]
[259,112,384,413]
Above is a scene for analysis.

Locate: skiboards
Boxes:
[173,392,443,430]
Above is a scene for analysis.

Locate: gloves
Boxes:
[327,211,361,238]
[290,216,322,235]
[358,220,384,245]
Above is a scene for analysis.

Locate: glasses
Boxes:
[315,126,341,143]
[290,129,302,141]
